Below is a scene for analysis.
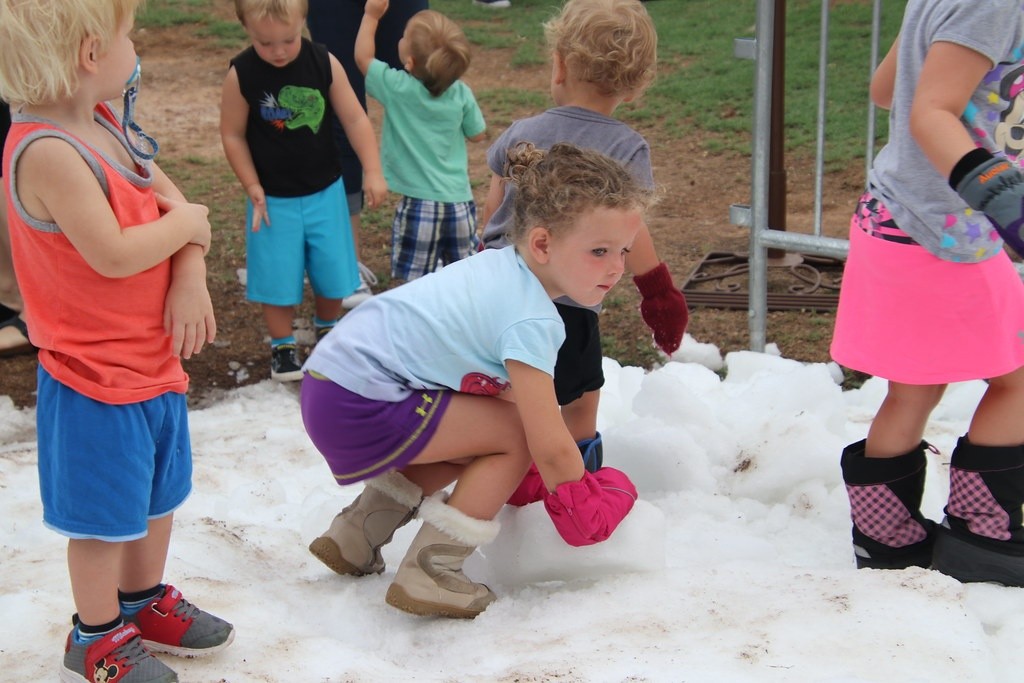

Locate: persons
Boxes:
[300,141,666,619]
[486,0,689,474]
[830,0,1024,588]
[354,0,487,283]
[0,0,235,683]
[221,0,390,381]
[305,0,430,308]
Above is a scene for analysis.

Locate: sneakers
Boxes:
[121,584,235,659]
[59,614,179,683]
[316,326,334,343]
[270,343,304,382]
[341,261,378,310]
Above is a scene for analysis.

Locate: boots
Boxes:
[309,468,428,577]
[385,489,498,618]
[576,431,603,473]
[931,431,1024,588]
[841,438,940,570]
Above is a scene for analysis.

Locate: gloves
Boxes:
[478,243,484,252]
[543,467,638,547]
[632,262,689,356]
[506,463,550,507]
[949,148,1024,260]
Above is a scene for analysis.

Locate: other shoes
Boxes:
[0,315,40,359]
[0,303,19,324]
